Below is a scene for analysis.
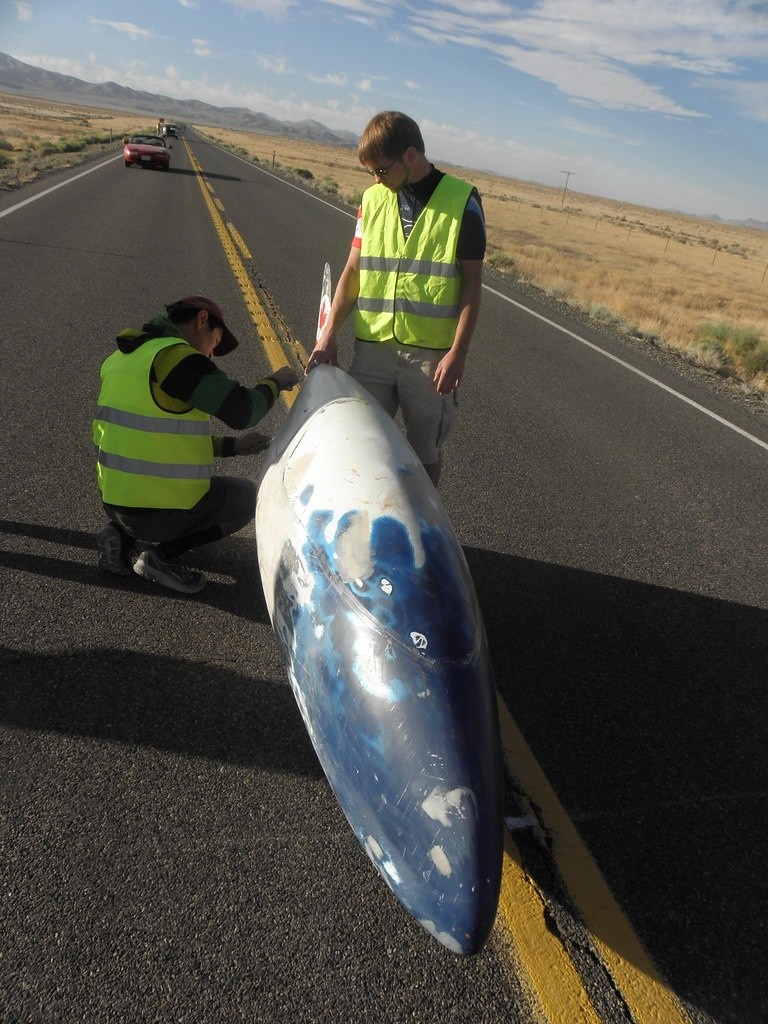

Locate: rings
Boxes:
[447,385,451,387]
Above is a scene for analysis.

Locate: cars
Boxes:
[121,134,173,169]
[163,124,180,139]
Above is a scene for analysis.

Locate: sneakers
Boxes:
[132,547,208,593]
[95,526,132,575]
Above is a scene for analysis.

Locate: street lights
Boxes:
[560,170,576,208]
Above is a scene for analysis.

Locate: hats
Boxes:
[164,295,239,356]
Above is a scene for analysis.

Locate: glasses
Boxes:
[365,145,410,176]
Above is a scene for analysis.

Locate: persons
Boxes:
[157,118,167,138]
[90,296,299,594]
[309,111,488,488]
